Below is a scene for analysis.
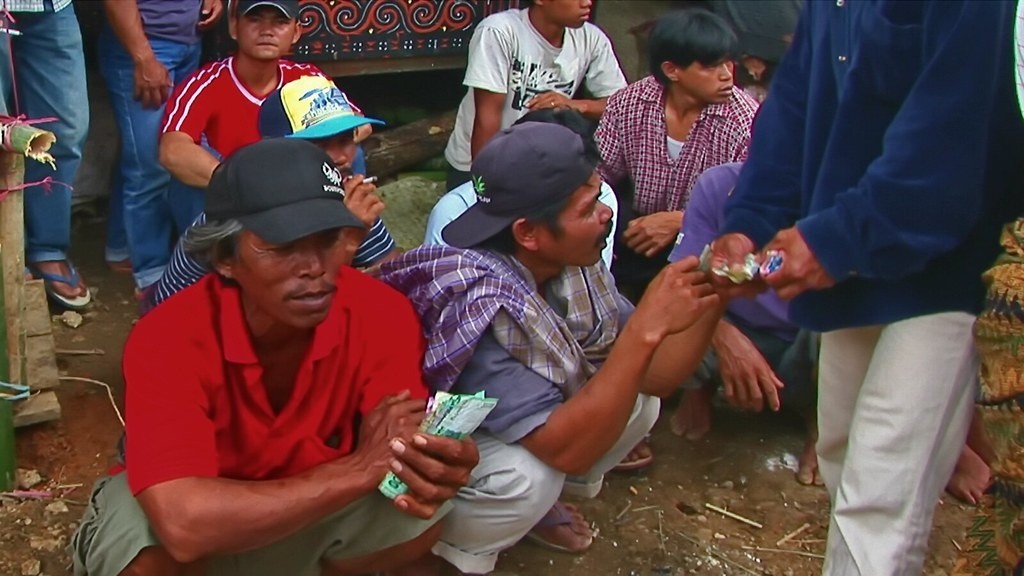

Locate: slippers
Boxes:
[615,442,656,470]
[526,506,592,555]
[33,258,92,310]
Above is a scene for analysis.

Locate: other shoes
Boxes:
[107,259,133,273]
[134,285,157,301]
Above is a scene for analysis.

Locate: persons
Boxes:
[422,0,994,507]
[0,0,404,308]
[64,137,480,576]
[421,122,770,576]
[709,0,1024,576]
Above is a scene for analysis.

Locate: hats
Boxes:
[203,137,366,242]
[235,0,299,19]
[258,76,386,139]
[442,121,595,248]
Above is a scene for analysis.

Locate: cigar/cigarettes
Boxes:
[362,176,378,184]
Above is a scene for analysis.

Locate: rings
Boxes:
[551,102,554,105]
